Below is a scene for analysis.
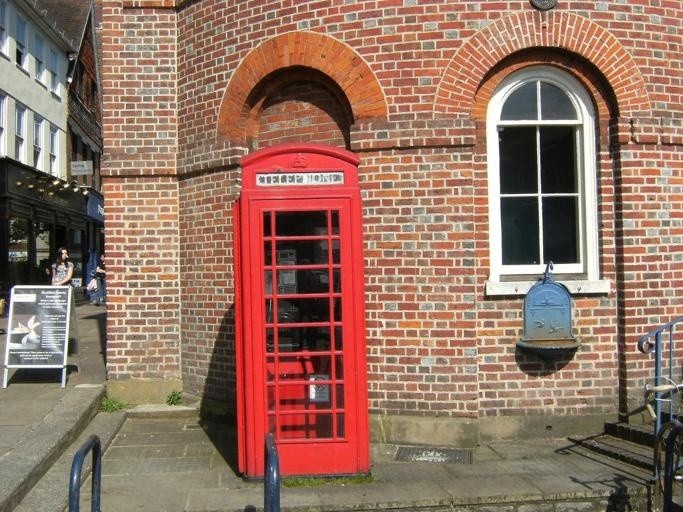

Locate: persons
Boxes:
[50,246,75,286]
[95,254,105,305]
